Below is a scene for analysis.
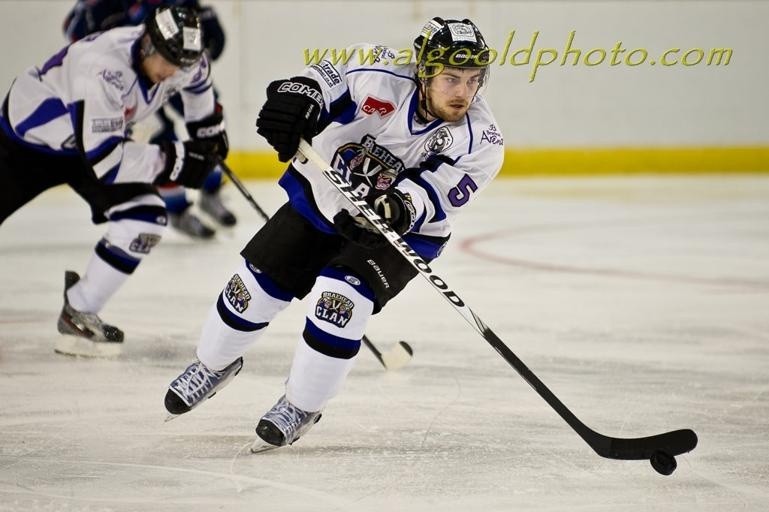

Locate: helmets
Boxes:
[148,4,204,67]
[411,16,489,82]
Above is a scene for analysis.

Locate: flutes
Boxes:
[216,156,413,372]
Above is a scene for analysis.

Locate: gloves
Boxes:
[189,104,234,158]
[257,74,325,160]
[151,140,208,190]
[331,190,412,248]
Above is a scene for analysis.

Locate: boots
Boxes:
[193,183,238,227]
[165,203,215,238]
[58,267,126,342]
[255,393,323,449]
[164,352,245,414]
[219,372,230,378]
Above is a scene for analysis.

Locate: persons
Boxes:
[164,16,505,447]
[0,4,228,345]
[62,0,237,239]
